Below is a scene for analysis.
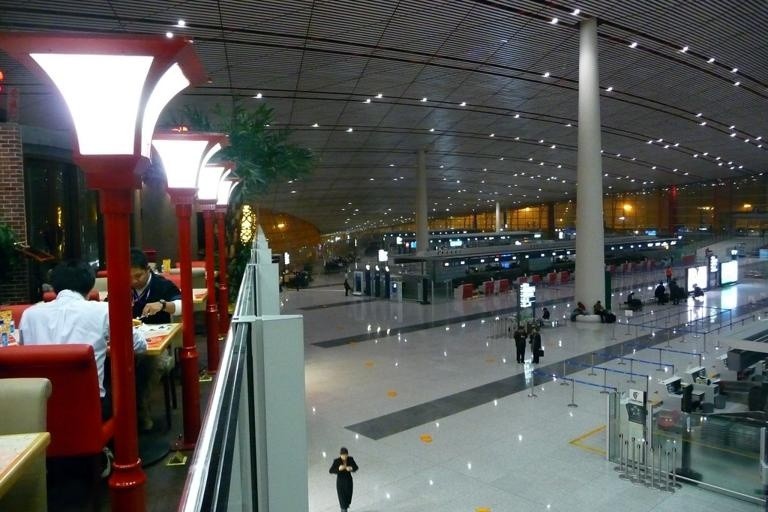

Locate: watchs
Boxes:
[160,299,167,312]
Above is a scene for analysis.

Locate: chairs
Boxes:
[176,261,208,338]
[0,377,54,512]
[453,254,696,301]
[1,344,114,511]
[155,272,183,430]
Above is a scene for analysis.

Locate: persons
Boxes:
[16,257,149,461]
[569,263,707,324]
[540,307,550,327]
[343,279,350,296]
[528,326,542,365]
[327,445,360,512]
[513,325,527,364]
[103,247,182,411]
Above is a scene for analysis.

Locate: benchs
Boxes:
[0,305,33,330]
